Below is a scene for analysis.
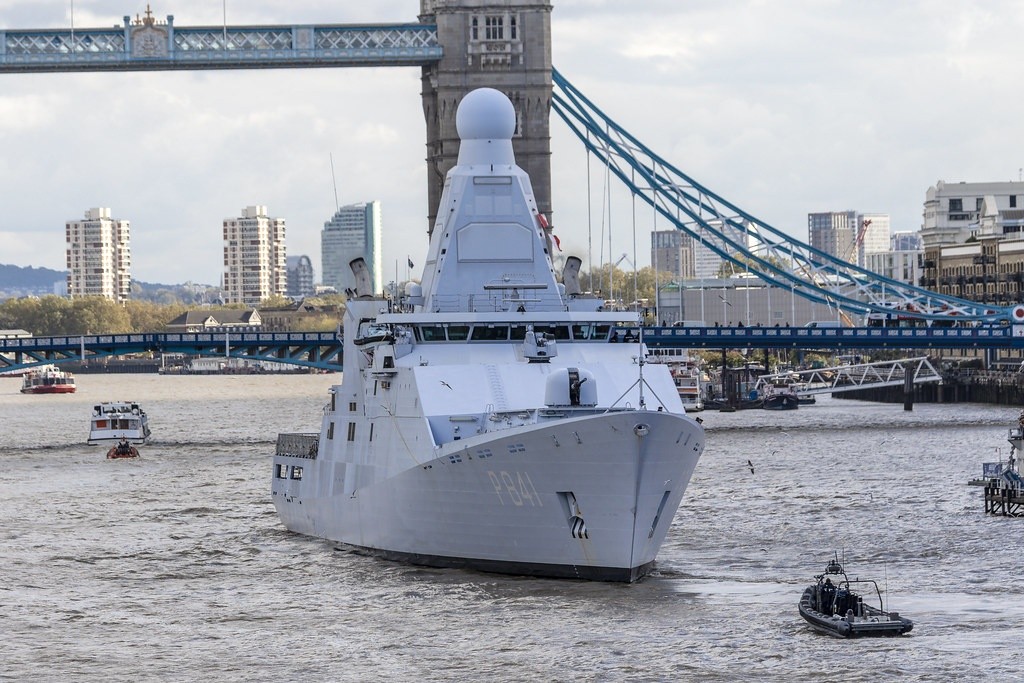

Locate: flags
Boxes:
[408,258,414,269]
[537,213,562,253]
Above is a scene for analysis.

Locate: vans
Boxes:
[800,320,840,336]
[670,320,707,336]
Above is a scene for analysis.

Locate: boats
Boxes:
[265,84,711,588]
[106,433,141,461]
[86,400,152,449]
[19,363,77,394]
[797,549,915,640]
